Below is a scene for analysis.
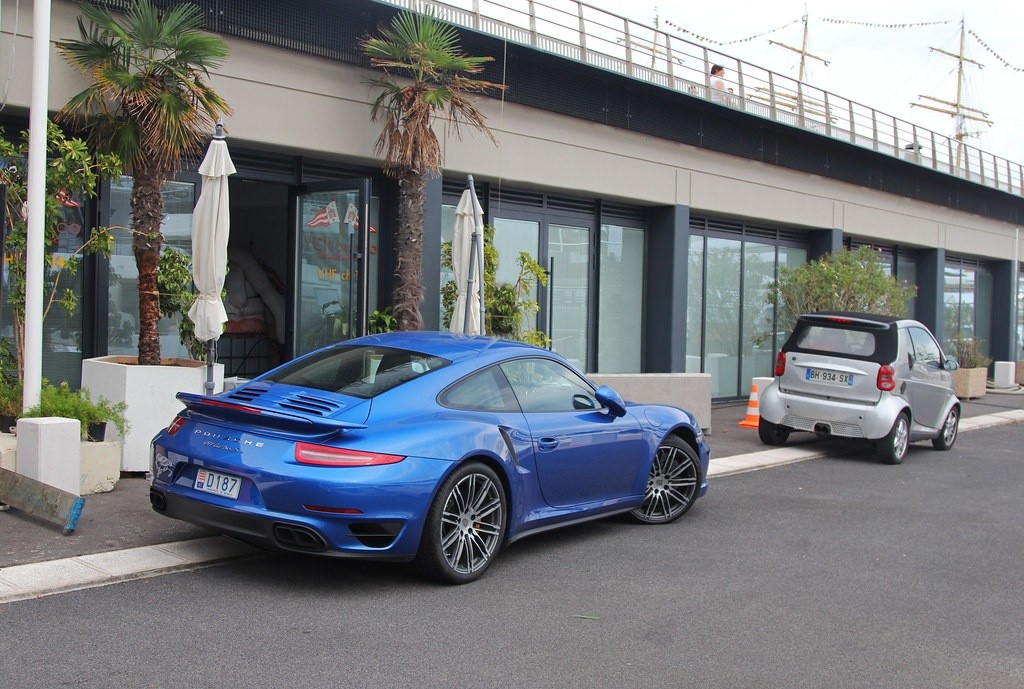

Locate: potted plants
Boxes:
[55,0,226,474]
[951,336,993,398]
[81,389,133,444]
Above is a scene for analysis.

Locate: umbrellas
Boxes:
[191,122,238,395]
[451,174,491,338]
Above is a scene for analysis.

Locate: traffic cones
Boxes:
[738,381,762,429]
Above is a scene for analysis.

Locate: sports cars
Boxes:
[145,327,714,586]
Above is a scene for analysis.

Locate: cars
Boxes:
[756,309,963,465]
